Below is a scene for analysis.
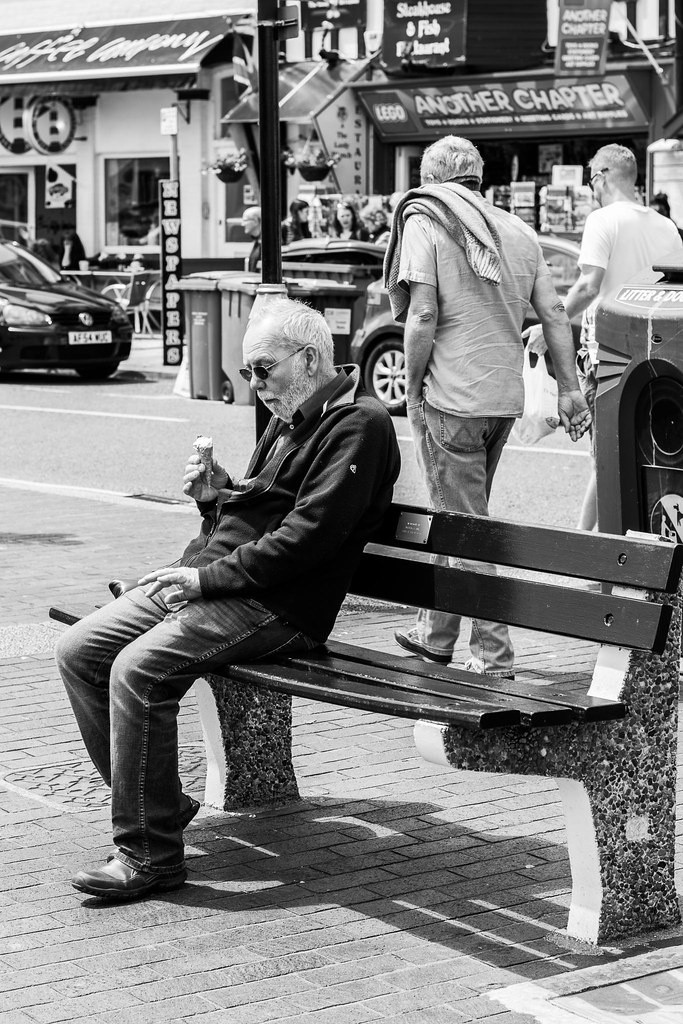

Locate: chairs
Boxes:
[114,271,152,336]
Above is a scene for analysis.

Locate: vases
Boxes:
[297,168,332,182]
[213,168,245,184]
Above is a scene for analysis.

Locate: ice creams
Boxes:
[193,435,213,488]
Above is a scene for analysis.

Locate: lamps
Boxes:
[318,20,340,67]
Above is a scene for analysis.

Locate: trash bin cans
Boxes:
[173,269,260,402]
[218,276,263,406]
[285,278,364,376]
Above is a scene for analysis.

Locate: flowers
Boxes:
[284,147,340,169]
[198,145,248,173]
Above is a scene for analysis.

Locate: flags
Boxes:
[45,162,73,208]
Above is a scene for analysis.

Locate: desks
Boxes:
[61,270,163,333]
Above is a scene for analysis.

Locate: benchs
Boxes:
[50,502,683,948]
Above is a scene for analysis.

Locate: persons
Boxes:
[241,200,392,273]
[392,136,594,682]
[50,223,86,270]
[56,297,402,900]
[521,143,683,532]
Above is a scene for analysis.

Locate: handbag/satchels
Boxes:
[514,345,560,447]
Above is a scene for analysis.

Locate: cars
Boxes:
[350,235,581,417]
[0,238,134,382]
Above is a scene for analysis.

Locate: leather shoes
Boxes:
[72,858,188,898]
[178,795,200,829]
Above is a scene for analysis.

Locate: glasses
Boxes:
[238,347,305,382]
[587,168,607,191]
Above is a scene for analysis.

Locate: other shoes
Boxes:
[394,631,452,666]
